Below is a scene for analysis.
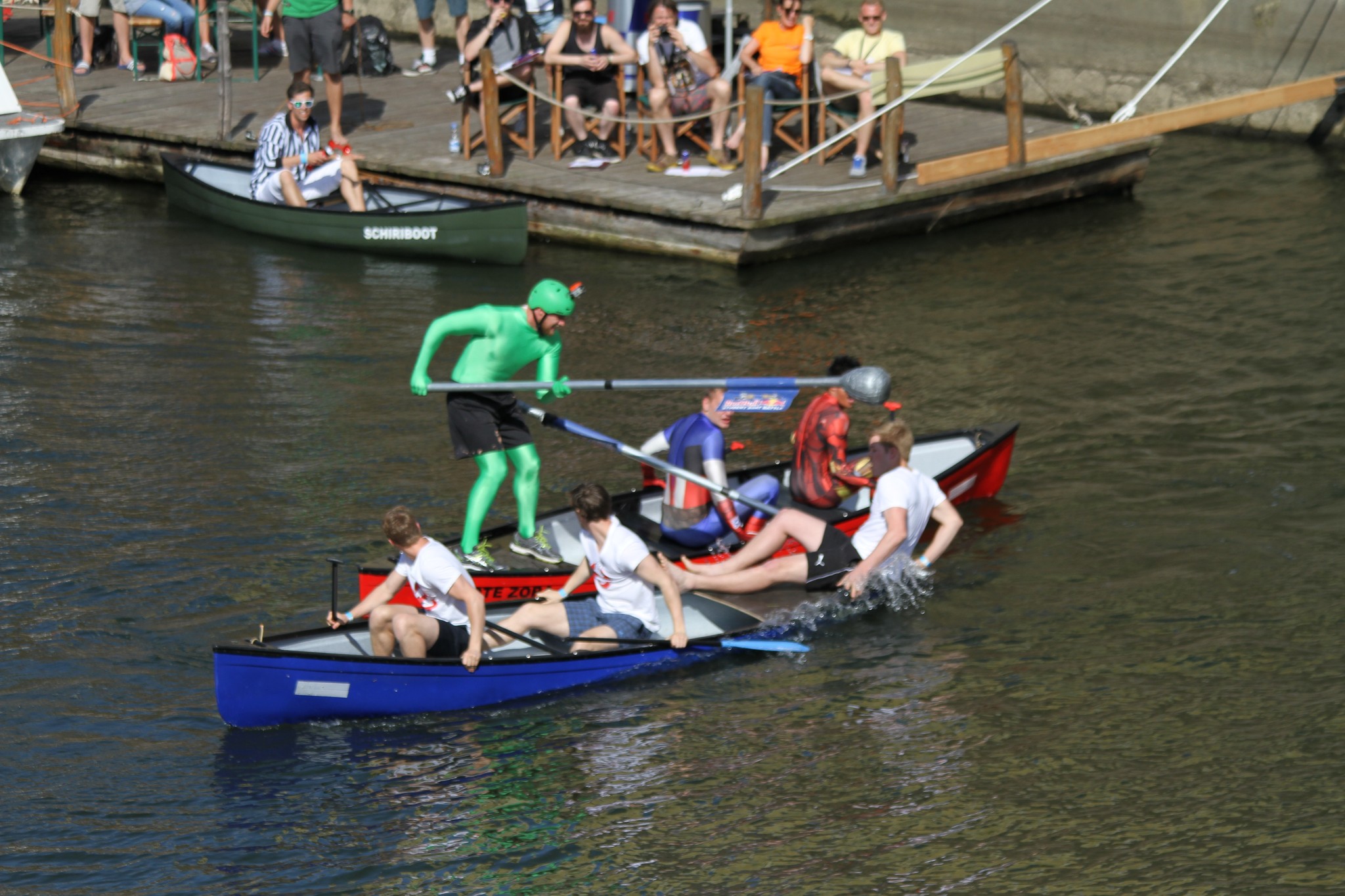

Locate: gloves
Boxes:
[552,376,571,398]
[733,527,757,542]
[410,364,432,396]
[641,476,665,488]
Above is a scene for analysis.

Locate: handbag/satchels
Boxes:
[159,33,196,81]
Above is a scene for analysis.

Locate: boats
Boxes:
[1,61,66,198]
[211,576,881,731]
[159,146,528,267]
[358,420,1025,618]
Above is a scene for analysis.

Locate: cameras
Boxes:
[657,25,668,34]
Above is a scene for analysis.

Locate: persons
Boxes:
[410,278,578,570]
[790,355,897,509]
[327,501,487,673]
[249,81,368,212]
[636,385,781,549]
[254,0,291,57]
[479,483,688,663]
[185,0,220,69]
[258,0,356,148]
[72,0,149,75]
[401,0,471,79]
[446,0,905,177]
[657,418,965,598]
[123,0,197,64]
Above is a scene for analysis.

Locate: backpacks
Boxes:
[342,15,394,77]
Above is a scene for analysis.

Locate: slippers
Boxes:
[73,61,90,77]
[119,58,149,73]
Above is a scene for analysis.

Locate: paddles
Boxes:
[720,440,747,456]
[565,628,812,657]
[884,401,902,424]
[324,559,342,628]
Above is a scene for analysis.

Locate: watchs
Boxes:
[342,9,355,15]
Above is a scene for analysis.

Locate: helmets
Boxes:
[528,279,575,316]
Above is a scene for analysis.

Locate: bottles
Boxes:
[448,124,460,157]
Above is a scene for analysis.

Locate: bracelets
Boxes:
[344,610,354,624]
[300,153,309,163]
[264,10,274,17]
[802,35,815,41]
[558,588,568,599]
[919,554,932,569]
[681,46,690,56]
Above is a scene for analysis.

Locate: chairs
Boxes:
[462,34,890,168]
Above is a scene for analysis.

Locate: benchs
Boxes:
[40,0,163,83]
[614,466,858,563]
[311,193,346,210]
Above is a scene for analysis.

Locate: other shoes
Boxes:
[850,152,867,179]
[446,86,467,104]
[200,43,218,63]
[707,148,737,171]
[578,139,621,164]
[460,64,464,72]
[403,60,438,77]
[646,150,679,171]
[258,42,289,57]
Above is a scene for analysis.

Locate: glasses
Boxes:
[290,98,315,109]
[574,10,591,15]
[785,8,801,14]
[862,15,880,21]
[493,0,511,3]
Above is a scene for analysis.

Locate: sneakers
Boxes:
[452,535,510,573]
[509,525,564,564]
